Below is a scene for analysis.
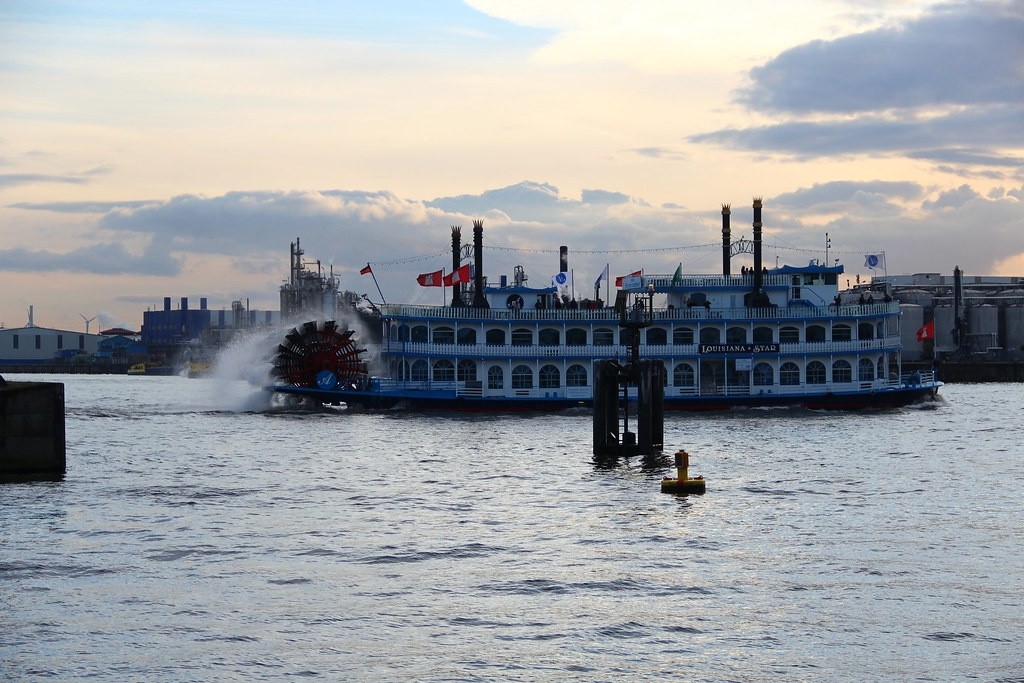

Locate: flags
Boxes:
[443,263,470,287]
[672,264,683,285]
[417,270,442,288]
[594,266,608,289]
[615,270,644,286]
[917,321,933,342]
[360,266,371,275]
[865,253,886,269]
[551,271,573,286]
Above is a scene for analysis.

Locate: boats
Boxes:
[262,195,946,417]
[660,450,706,496]
[125,356,221,379]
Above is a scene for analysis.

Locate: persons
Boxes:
[535,298,604,319]
[741,266,769,285]
[687,297,711,311]
[834,292,892,315]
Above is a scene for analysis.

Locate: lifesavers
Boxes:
[828,303,836,312]
[870,390,875,396]
